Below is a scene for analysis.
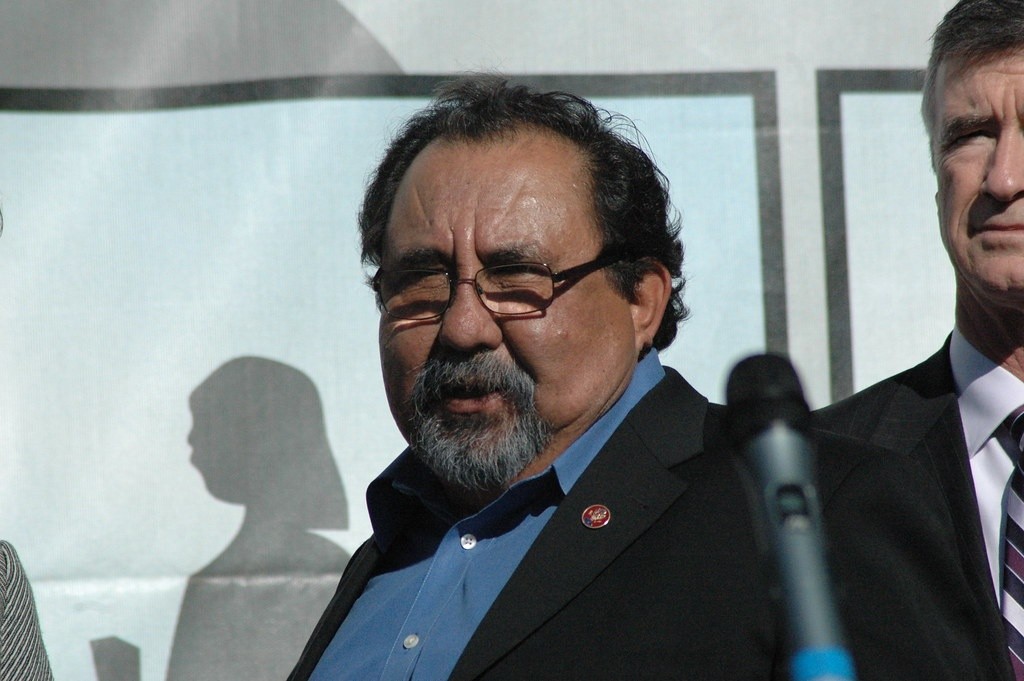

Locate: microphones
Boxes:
[725,353,857,681]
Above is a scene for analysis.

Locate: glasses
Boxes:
[371,246,634,320]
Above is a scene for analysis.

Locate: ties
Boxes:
[1003,404,1024,681]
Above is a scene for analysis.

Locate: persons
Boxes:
[286,75,796,681]
[797,0,1023,681]
